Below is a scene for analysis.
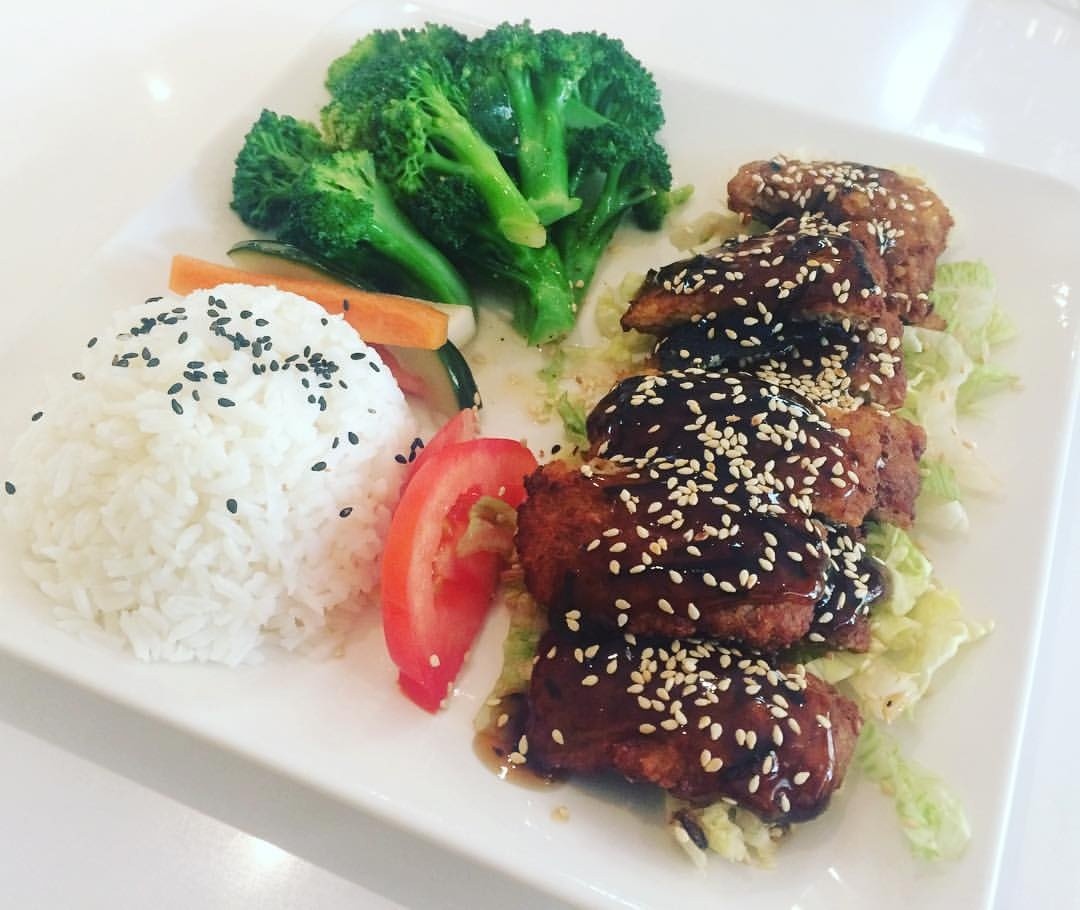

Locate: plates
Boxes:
[2,4,1077,909]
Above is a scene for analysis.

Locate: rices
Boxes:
[1,268,434,682]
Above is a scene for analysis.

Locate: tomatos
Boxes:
[391,407,478,507]
[375,435,550,714]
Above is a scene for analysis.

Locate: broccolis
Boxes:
[222,7,678,355]
